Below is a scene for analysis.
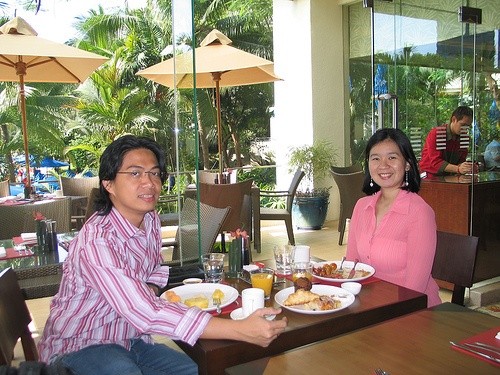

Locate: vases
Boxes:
[34,221,52,255]
[225,237,249,276]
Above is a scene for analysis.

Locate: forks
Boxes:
[213,299,221,313]
[375,368,385,375]
[336,256,346,274]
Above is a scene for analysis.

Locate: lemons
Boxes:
[212,288,224,299]
[184,296,208,307]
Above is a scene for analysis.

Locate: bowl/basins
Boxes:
[340,282,362,295]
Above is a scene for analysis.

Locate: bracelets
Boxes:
[457,165,459,173]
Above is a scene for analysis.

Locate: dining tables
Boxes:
[226,301,500,375]
[0,227,82,298]
[167,255,428,374]
[1,195,87,230]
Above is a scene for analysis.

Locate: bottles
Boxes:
[44,220,58,254]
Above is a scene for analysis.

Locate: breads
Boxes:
[282,289,321,306]
[294,277,312,291]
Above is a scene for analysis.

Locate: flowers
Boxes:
[33,211,44,222]
[228,225,247,239]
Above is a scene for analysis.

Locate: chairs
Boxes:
[0,164,478,375]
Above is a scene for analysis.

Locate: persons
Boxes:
[35,135,289,375]
[483,130,500,171]
[418,107,477,178]
[344,128,443,309]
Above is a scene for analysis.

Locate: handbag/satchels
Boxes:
[167,264,205,283]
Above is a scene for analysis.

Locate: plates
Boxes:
[297,262,316,269]
[274,284,356,315]
[182,277,203,284]
[306,260,376,283]
[159,282,239,312]
[229,306,277,321]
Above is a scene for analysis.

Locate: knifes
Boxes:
[450,340,500,363]
[347,258,360,279]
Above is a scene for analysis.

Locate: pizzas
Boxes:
[314,263,338,276]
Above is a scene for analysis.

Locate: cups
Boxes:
[241,287,265,318]
[249,267,275,300]
[293,245,310,263]
[200,253,225,283]
[466,153,472,162]
[290,261,313,292]
[273,244,297,276]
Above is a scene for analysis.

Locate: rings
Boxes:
[277,335,279,337]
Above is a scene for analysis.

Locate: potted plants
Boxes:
[291,137,343,232]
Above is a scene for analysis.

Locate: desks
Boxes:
[417,170,500,291]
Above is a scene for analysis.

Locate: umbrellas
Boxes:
[135,28,285,184]
[31,157,70,177]
[375,64,388,110]
[0,18,109,191]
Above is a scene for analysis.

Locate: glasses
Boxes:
[116,166,164,179]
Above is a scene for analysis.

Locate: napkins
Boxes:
[0,246,35,260]
[13,232,38,247]
[1,200,27,207]
[449,327,500,367]
[59,240,72,252]
